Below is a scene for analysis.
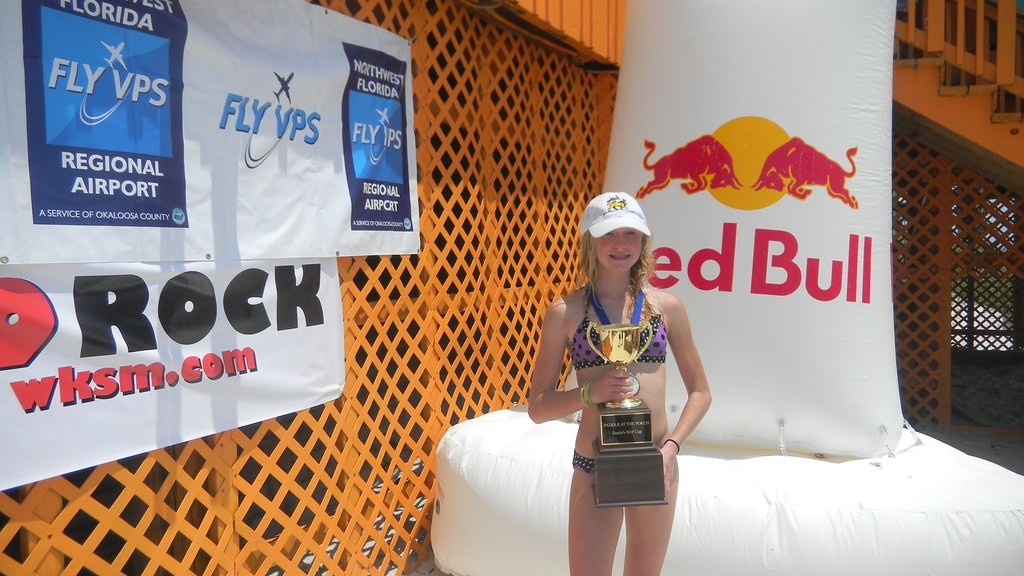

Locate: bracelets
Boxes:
[664,439,680,454]
[581,383,598,408]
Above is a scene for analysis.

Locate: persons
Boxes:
[527,192,711,576]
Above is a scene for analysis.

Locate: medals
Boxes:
[629,375,640,396]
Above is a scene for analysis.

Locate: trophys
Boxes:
[586,319,668,507]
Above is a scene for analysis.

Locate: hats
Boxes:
[579,191,651,239]
